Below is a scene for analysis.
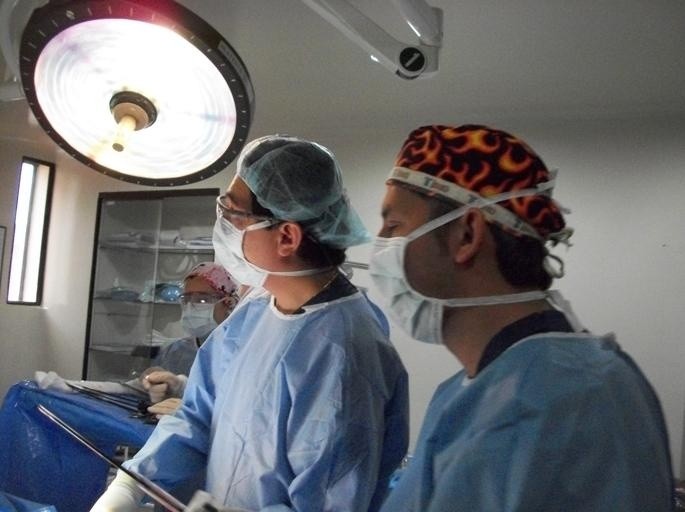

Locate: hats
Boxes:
[392,121,563,245]
[185,260,239,307]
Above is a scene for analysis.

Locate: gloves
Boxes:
[143,372,187,404]
[88,471,145,512]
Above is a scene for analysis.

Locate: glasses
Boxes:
[181,292,225,304]
[213,196,271,223]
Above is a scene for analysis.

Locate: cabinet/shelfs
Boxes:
[82,189,220,383]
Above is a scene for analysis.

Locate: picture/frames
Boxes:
[0,225,8,288]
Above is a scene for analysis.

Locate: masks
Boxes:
[180,298,233,336]
[363,186,600,342]
[209,210,333,289]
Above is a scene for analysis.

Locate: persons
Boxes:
[86,132,411,511]
[141,369,195,422]
[370,121,677,511]
[135,257,241,390]
